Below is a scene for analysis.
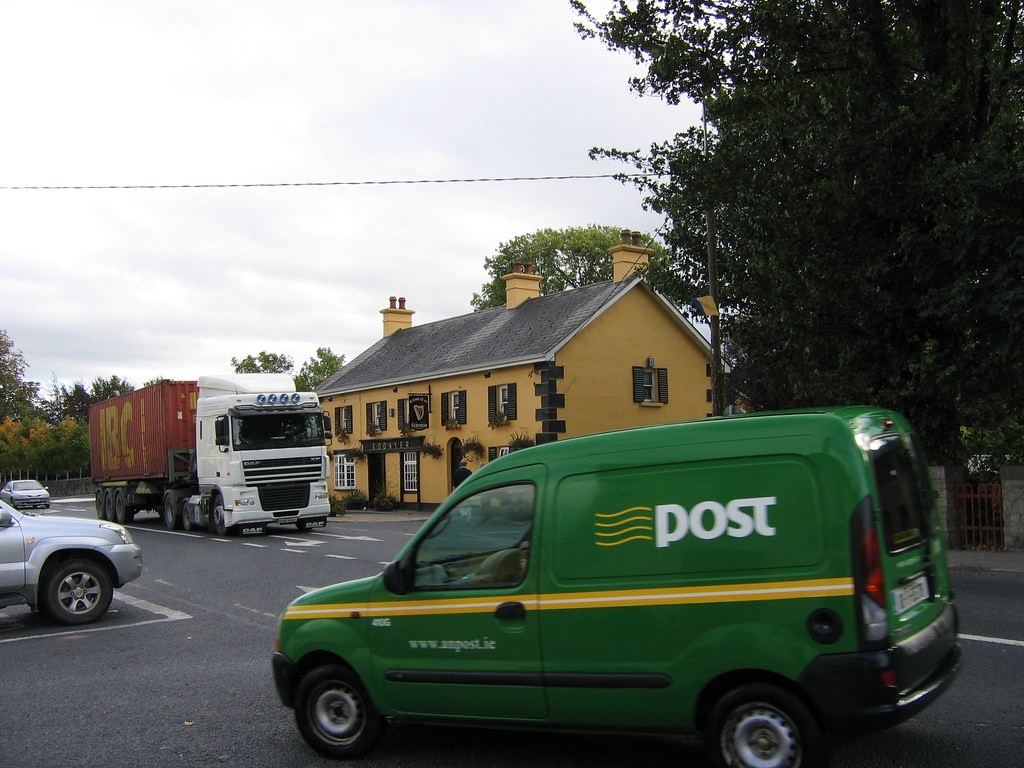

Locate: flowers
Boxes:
[335,427,349,445]
[444,419,461,432]
[458,435,484,462]
[506,427,535,451]
[421,441,444,460]
[345,447,364,464]
[365,422,380,438]
[401,422,411,439]
[488,409,511,430]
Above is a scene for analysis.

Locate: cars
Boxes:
[0,479,51,510]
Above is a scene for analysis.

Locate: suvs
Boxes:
[0,499,143,627]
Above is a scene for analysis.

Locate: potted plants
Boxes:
[329,478,396,518]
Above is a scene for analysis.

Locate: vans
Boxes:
[267,402,968,768]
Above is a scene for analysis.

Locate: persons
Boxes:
[454,459,493,524]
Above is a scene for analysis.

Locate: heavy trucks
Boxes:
[86,371,334,539]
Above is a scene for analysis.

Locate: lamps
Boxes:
[484,371,490,378]
[393,386,397,393]
[328,395,332,402]
